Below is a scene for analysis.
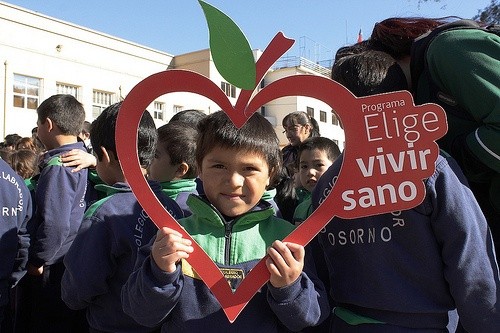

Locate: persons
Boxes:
[0,17,500,333]
[121,107,331,333]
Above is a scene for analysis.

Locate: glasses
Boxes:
[281,125,304,136]
